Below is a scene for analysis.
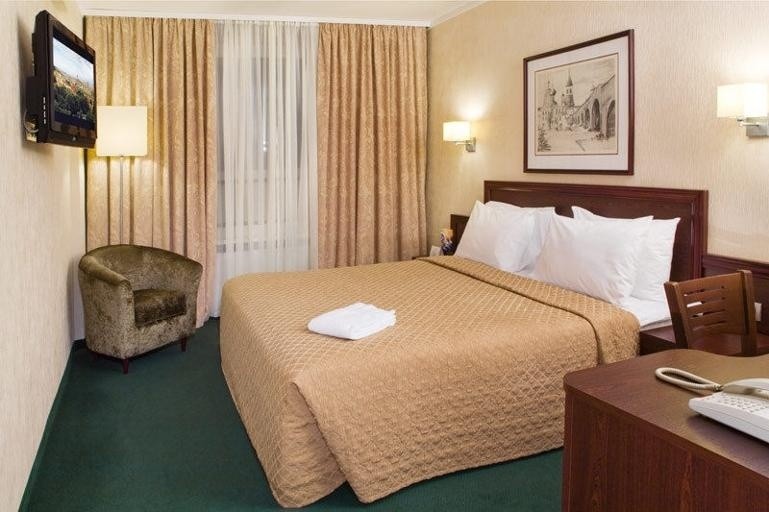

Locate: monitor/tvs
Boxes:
[26,10,98,151]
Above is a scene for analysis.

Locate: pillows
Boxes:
[529,212,654,303]
[485,199,554,272]
[454,200,537,272]
[571,206,681,301]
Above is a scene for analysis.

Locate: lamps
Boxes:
[441,120,475,151]
[715,81,769,135]
[96,105,149,245]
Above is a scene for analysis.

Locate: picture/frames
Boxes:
[523,29,635,175]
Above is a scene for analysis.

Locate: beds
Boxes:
[219,181,708,510]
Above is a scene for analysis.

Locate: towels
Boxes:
[307,300,396,340]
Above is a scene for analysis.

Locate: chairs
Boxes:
[78,244,202,372]
[664,269,757,355]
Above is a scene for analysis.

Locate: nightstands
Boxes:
[412,256,429,260]
[640,319,769,356]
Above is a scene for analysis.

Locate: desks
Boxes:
[561,333,768,512]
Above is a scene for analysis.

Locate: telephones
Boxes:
[688,378,769,444]
